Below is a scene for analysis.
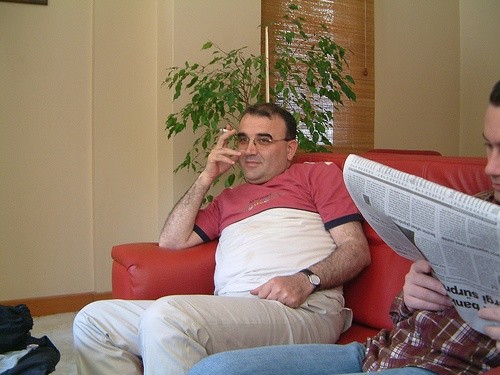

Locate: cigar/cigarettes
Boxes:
[219,128,230,133]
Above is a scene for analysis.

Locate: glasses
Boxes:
[234,136,291,145]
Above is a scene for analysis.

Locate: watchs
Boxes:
[300,269,321,294]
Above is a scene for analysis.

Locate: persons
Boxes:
[72,103,370,375]
[187,80,500,375]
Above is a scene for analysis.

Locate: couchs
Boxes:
[111,150,500,375]
